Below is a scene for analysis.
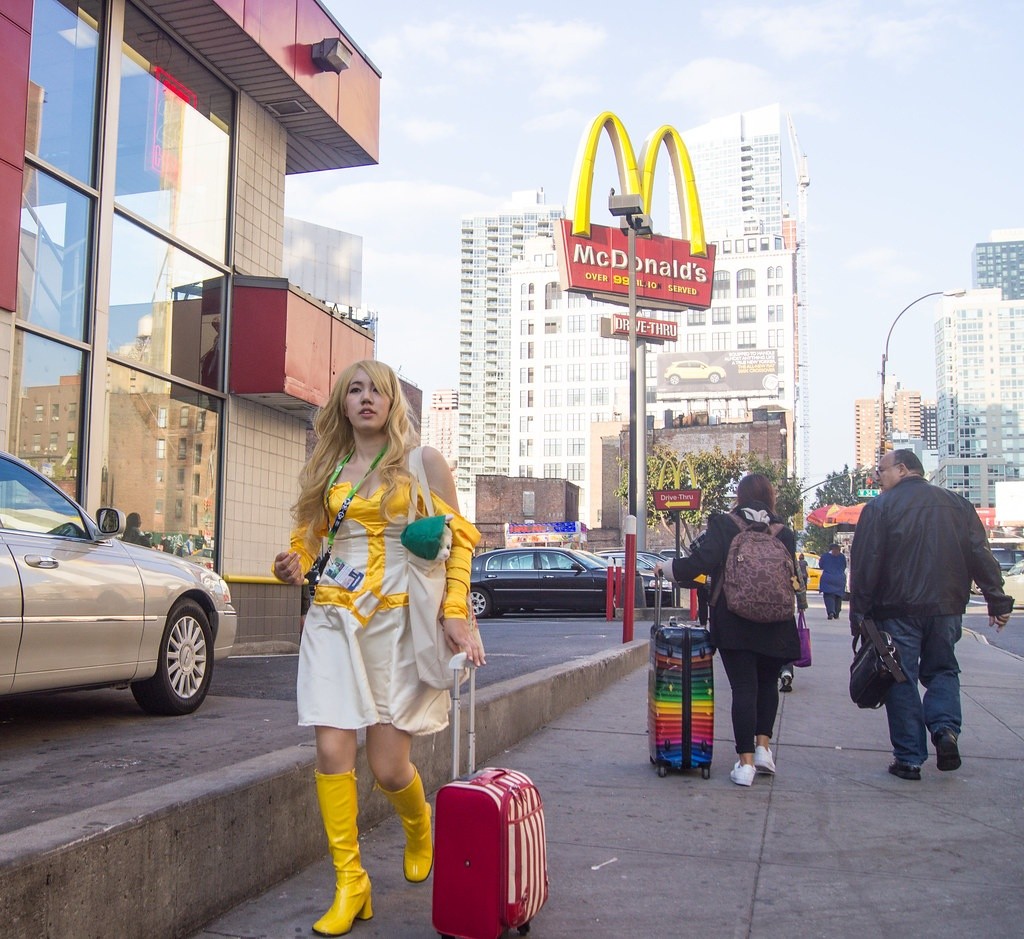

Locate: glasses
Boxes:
[876,461,902,478]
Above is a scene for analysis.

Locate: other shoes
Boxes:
[827,613,833,619]
[779,670,793,692]
[835,615,840,618]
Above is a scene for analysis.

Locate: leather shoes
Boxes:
[934,729,962,771]
[889,758,922,779]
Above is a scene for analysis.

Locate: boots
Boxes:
[376,761,432,882]
[313,768,373,936]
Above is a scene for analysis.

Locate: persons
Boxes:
[654,474,803,786]
[124,513,214,556]
[819,544,847,619]
[798,553,809,590]
[850,449,1015,779]
[275,361,486,937]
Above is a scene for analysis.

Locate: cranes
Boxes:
[787,113,810,245]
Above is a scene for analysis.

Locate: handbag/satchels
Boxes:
[792,610,812,668]
[850,621,906,708]
[400,447,476,689]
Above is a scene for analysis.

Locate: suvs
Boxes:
[664,360,727,386]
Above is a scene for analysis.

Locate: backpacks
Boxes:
[723,512,795,624]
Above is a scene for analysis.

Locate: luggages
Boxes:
[648,568,715,778]
[430,651,548,939]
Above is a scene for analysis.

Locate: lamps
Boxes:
[312,38,353,75]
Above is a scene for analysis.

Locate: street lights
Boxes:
[880,288,967,456]
[606,189,654,517]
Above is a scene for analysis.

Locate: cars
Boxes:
[182,548,215,571]
[971,549,1024,595]
[468,546,673,618]
[796,551,822,590]
[0,447,238,715]
[1001,557,1024,607]
[569,548,685,575]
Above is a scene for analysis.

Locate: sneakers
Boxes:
[730,760,758,786]
[755,746,776,773]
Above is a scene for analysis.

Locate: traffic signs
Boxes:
[654,488,702,511]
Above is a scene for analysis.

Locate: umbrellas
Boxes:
[807,502,847,527]
[825,502,866,524]
